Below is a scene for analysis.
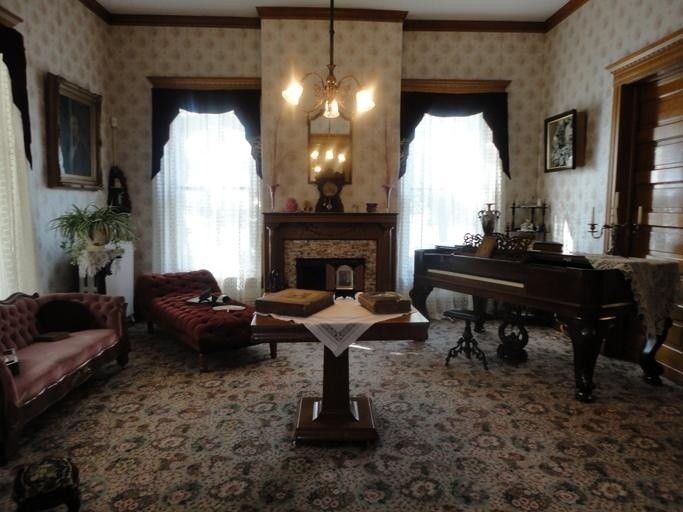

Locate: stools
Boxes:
[442,307,491,372]
[13,457,82,512]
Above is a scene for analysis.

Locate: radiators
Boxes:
[77,240,135,326]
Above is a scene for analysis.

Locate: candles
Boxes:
[589,192,643,225]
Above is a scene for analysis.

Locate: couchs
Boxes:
[1,292,129,459]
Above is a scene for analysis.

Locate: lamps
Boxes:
[282,0,375,121]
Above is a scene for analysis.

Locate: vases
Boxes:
[477,203,501,235]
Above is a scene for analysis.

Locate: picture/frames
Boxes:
[45,70,104,190]
[543,108,585,173]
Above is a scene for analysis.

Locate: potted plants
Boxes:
[47,204,142,266]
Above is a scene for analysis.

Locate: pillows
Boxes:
[35,299,94,334]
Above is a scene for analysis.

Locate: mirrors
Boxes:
[308,109,353,185]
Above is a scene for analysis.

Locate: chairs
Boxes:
[138,269,281,370]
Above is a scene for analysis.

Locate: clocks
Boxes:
[312,168,346,211]
